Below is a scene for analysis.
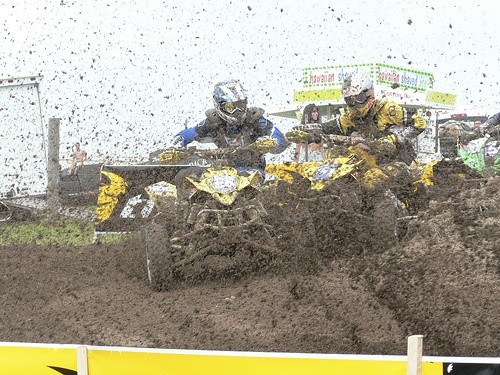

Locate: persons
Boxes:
[292,102,332,164]
[282,69,427,221]
[168,79,288,199]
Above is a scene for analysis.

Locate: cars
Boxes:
[53,162,109,207]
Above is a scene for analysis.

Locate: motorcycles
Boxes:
[292,124,428,254]
[136,145,318,287]
[436,133,500,258]
